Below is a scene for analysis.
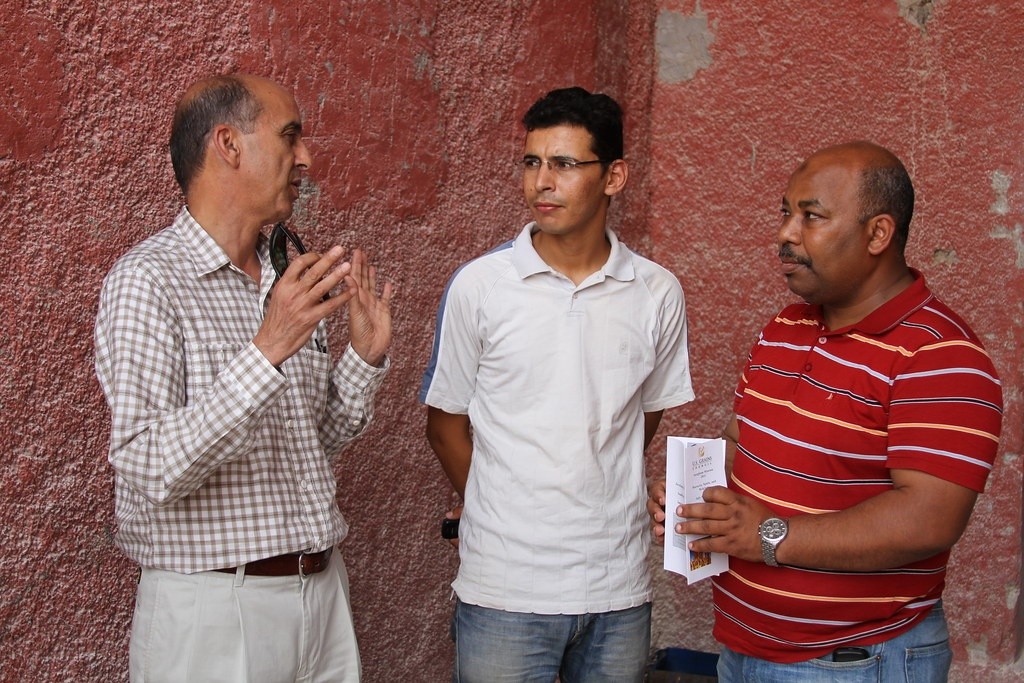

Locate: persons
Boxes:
[648,141,1007,683]
[412,86,698,681]
[90,71,395,683]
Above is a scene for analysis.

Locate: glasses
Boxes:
[513,152,614,173]
[270,222,331,308]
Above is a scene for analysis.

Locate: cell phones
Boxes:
[832,647,870,662]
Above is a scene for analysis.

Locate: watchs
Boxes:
[760,514,788,568]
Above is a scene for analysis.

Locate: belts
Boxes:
[211,545,338,576]
